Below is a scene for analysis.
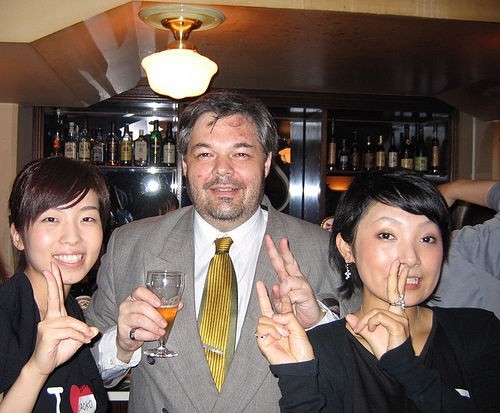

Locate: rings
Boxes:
[131,289,135,301]
[391,295,404,308]
[129,327,140,340]
[255,331,267,339]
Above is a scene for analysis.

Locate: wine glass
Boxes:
[144,269,185,358]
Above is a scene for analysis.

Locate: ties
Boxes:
[197,236,238,394]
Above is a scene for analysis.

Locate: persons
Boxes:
[255,166,500,413]
[80,90,363,413]
[422,179,500,320]
[0,154,110,413]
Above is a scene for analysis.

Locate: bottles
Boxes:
[46,116,177,167]
[327,117,450,176]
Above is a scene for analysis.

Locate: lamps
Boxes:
[137,4,226,100]
